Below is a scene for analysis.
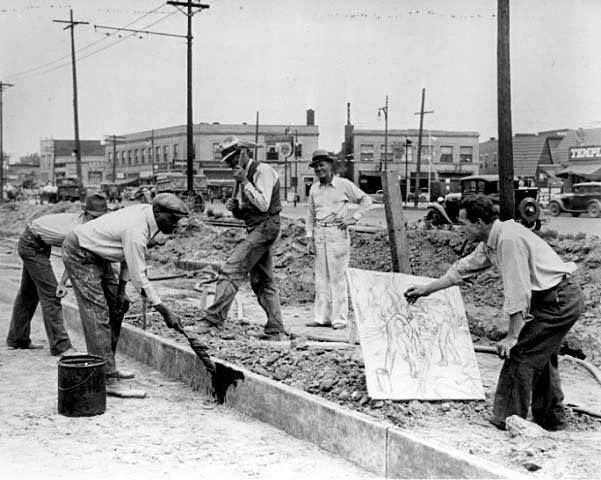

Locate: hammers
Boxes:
[232,141,264,199]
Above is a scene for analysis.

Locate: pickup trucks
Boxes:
[365,189,384,201]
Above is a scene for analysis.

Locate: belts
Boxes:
[316,221,341,226]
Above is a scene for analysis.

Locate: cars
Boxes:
[424,174,541,232]
[407,193,426,204]
[549,182,601,219]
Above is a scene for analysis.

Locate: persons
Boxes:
[305,150,373,330]
[5,195,110,357]
[61,193,189,398]
[403,193,585,431]
[181,136,286,341]
[39,179,58,204]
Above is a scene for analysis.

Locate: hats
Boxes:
[153,192,190,218]
[218,135,243,163]
[309,149,334,167]
[81,194,107,217]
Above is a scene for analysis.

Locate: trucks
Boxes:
[42,177,85,204]
[139,171,207,216]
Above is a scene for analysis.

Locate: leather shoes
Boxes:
[7,340,45,349]
[107,369,136,378]
[194,318,223,330]
[55,346,81,356]
[105,381,147,398]
[333,323,346,329]
[306,321,332,327]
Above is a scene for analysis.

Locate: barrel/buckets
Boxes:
[56,353,106,416]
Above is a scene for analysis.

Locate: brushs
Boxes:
[183,329,217,373]
